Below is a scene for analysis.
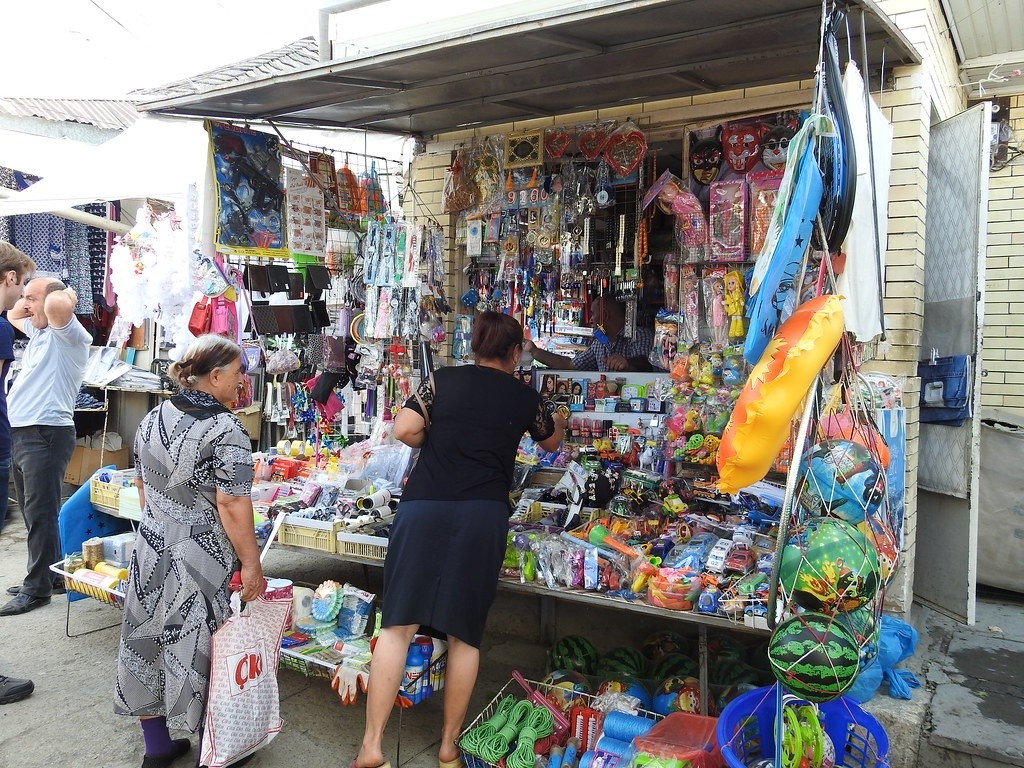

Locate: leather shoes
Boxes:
[7,577,66,596]
[0,592,51,616]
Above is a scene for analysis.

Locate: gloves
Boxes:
[331,657,369,708]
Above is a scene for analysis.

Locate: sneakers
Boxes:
[0,675,35,705]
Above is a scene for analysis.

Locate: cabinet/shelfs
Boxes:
[49,370,907,768]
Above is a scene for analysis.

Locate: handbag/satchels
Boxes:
[198,589,294,768]
[340,308,354,335]
[266,348,300,374]
[195,256,244,302]
[239,343,261,373]
[211,256,238,344]
[188,302,212,338]
[308,335,345,368]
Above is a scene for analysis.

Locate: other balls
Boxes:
[541,628,775,719]
[768,423,904,704]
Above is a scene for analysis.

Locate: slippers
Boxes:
[438,755,464,768]
[351,754,391,768]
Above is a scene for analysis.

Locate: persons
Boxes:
[722,267,761,337]
[114,333,263,768]
[660,181,706,245]
[665,264,678,311]
[708,280,726,349]
[683,280,699,345]
[655,322,678,372]
[753,189,778,254]
[540,375,581,395]
[353,311,567,768]
[0,241,93,706]
[513,370,532,385]
[521,291,656,373]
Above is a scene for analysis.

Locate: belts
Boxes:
[262,382,315,438]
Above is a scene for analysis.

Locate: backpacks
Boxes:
[229,373,253,410]
[336,166,389,215]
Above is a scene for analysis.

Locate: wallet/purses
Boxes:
[307,374,345,421]
[241,264,291,298]
[244,305,314,334]
[309,372,337,405]
[286,273,304,300]
[305,265,332,293]
[311,301,331,327]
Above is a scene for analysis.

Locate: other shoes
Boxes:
[195,751,256,768]
[141,738,191,768]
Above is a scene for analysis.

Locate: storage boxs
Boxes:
[63,444,129,485]
[539,373,556,394]
[521,367,535,388]
[710,180,748,261]
[90,478,124,510]
[748,178,781,262]
[232,402,263,440]
[557,378,572,394]
[572,379,589,400]
[278,516,347,554]
[337,512,396,559]
